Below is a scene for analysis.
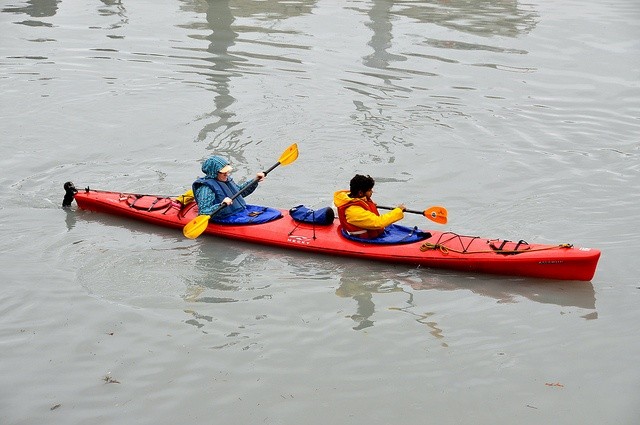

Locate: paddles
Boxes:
[374,205,447,225]
[183,142,299,240]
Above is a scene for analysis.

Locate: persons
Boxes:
[334,173,407,238]
[190,153,265,221]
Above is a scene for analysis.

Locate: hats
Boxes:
[218,165,231,173]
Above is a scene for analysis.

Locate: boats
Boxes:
[62,181,601,280]
